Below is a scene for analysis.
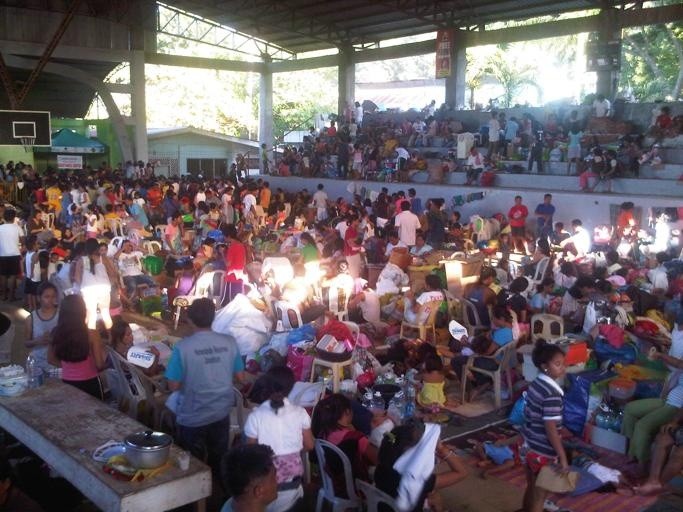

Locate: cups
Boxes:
[176,452,191,471]
[34,368,44,385]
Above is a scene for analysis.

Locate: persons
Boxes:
[0,96,683,512]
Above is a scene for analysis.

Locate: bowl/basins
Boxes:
[0,376,27,394]
[409,255,424,267]
[480,248,497,254]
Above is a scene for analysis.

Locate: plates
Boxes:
[92,441,126,462]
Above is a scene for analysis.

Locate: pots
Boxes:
[125,428,174,468]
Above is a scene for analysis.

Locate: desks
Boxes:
[0,375,212,512]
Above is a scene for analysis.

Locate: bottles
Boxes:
[595,401,623,432]
[26,351,40,389]
[363,371,416,427]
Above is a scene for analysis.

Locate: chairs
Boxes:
[529,312,566,344]
[523,255,551,297]
[41,212,55,229]
[469,215,490,244]
[460,339,516,410]
[274,301,303,331]
[313,438,363,512]
[320,272,354,321]
[99,224,169,296]
[103,343,166,420]
[399,290,444,342]
[505,261,520,281]
[310,320,360,395]
[354,477,399,512]
[458,296,490,341]
[172,268,226,330]
[227,385,256,450]
[24,224,28,236]
[443,288,463,322]
